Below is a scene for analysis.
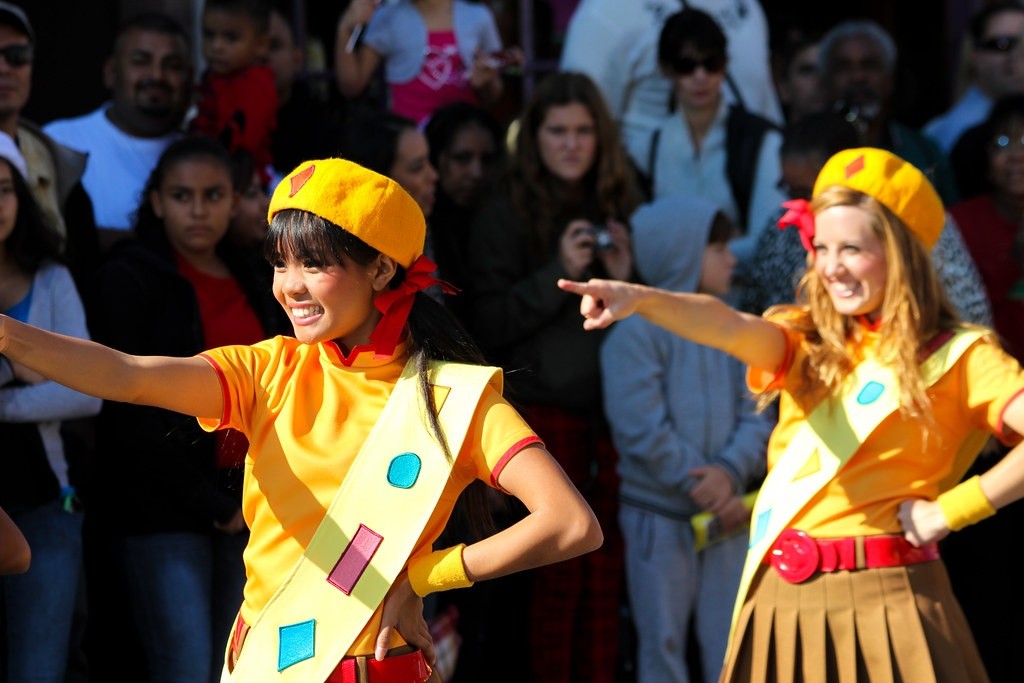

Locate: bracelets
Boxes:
[407,543,472,596]
[940,475,996,533]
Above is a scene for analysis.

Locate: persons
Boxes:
[1,158,604,681]
[741,0,1024,683]
[336,0,776,683]
[558,147,1024,682]
[0,0,345,682]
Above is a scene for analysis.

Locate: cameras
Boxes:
[581,226,615,254]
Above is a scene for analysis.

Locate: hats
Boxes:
[0,131,30,183]
[777,147,948,257]
[0,1,33,39]
[266,157,457,368]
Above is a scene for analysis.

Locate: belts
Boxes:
[756,528,938,585]
[227,614,432,683]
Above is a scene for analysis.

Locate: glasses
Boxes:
[975,34,1024,52]
[669,53,729,80]
[0,37,36,67]
